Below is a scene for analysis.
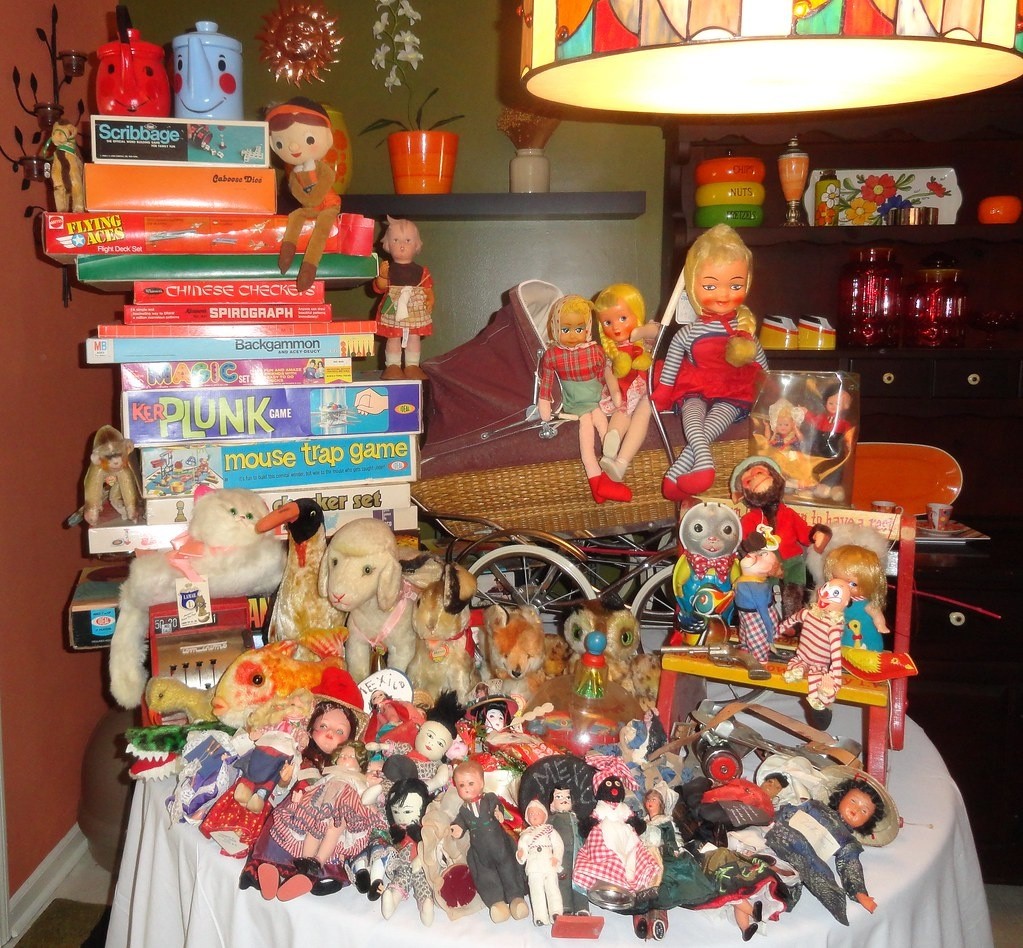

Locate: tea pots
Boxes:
[171,20,244,121]
[98,5,170,119]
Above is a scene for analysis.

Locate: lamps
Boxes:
[515,1,1023,126]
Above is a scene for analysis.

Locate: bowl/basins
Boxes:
[887,206,939,227]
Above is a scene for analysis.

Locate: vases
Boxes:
[387,128,460,194]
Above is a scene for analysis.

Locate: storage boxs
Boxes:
[39,114,424,654]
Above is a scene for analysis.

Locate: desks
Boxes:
[104,612,993,943]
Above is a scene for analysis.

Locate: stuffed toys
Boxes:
[266,95,340,292]
[729,456,829,636]
[84,423,138,523]
[107,485,662,789]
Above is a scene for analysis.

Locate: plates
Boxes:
[915,520,970,536]
[915,520,990,544]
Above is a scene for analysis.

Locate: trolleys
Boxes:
[424,280,862,663]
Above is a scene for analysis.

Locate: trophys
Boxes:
[776,136,810,228]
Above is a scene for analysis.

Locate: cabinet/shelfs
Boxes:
[654,122,1023,880]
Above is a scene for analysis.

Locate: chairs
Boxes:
[654,494,917,788]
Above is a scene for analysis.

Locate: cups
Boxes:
[870,500,903,517]
[925,503,953,530]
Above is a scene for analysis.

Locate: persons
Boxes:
[539,283,661,506]
[732,523,899,707]
[651,226,768,501]
[167,692,900,941]
[769,381,854,505]
[370,215,435,381]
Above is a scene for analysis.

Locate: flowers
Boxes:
[353,0,465,150]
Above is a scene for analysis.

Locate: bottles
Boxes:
[904,268,970,324]
[510,149,551,194]
[814,170,841,225]
[521,660,645,758]
[847,245,902,324]
